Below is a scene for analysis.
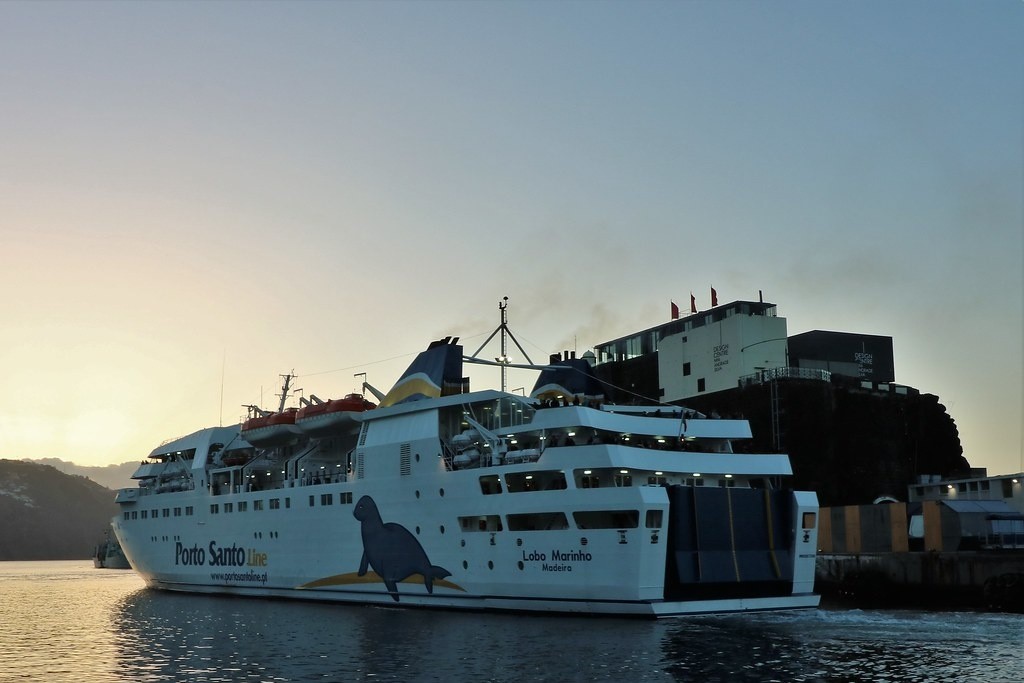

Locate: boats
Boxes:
[92,530,131,569]
[109,295,822,618]
